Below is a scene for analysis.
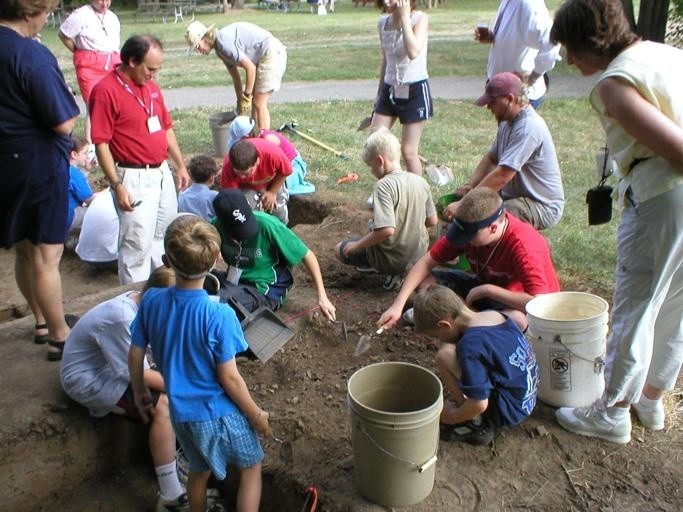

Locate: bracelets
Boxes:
[250,410,262,423]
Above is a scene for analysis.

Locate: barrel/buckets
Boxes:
[435,194,472,271]
[209,113,236,157]
[525,291,609,408]
[349,362,445,506]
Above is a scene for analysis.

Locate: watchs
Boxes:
[111,181,123,190]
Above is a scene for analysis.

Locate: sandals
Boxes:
[34,319,51,345]
[46,340,67,363]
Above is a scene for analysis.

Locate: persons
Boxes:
[549,0,683,445]
[59,265,223,512]
[65,133,97,253]
[58,0,122,110]
[374,187,562,334]
[209,187,337,325]
[352,0,447,11]
[182,20,287,130]
[177,154,220,225]
[0,0,82,361]
[88,33,191,287]
[225,115,316,195]
[333,125,438,293]
[412,281,539,446]
[366,1,434,208]
[74,185,120,273]
[128,213,273,512]
[443,70,564,231]
[473,0,561,115]
[221,137,293,229]
[258,0,337,16]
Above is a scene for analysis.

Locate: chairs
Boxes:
[46,0,63,28]
[143,0,154,22]
[150,0,160,23]
[133,0,145,23]
[161,0,175,24]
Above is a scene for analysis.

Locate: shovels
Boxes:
[219,108,243,133]
[326,310,350,344]
[276,121,352,164]
[352,318,382,357]
[417,153,455,186]
[356,102,376,132]
[267,427,296,471]
[228,296,297,367]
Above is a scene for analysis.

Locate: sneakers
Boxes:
[634,403,666,432]
[380,271,402,291]
[147,444,195,484]
[555,399,634,445]
[155,495,218,508]
[439,416,496,446]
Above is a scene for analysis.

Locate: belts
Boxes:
[115,160,162,169]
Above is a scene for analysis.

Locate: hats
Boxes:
[183,19,216,55]
[472,70,526,107]
[212,186,258,242]
[161,212,218,280]
[445,199,504,247]
[227,114,256,152]
